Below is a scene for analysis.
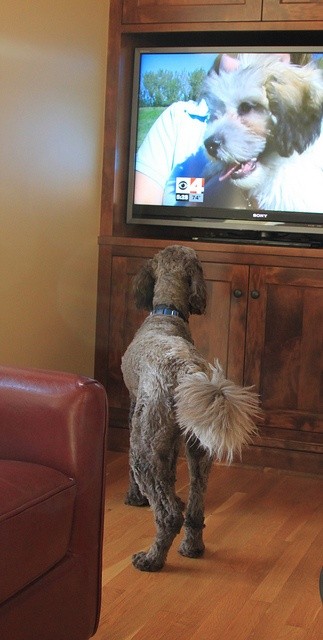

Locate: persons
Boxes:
[132,51,311,206]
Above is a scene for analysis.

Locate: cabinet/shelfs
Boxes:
[99,0,323,257]
[94,245,323,475]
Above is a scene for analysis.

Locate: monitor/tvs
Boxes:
[124,47,322,235]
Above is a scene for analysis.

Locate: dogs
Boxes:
[198,54,323,214]
[121,245,264,574]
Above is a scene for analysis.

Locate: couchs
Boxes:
[1,365,110,640]
